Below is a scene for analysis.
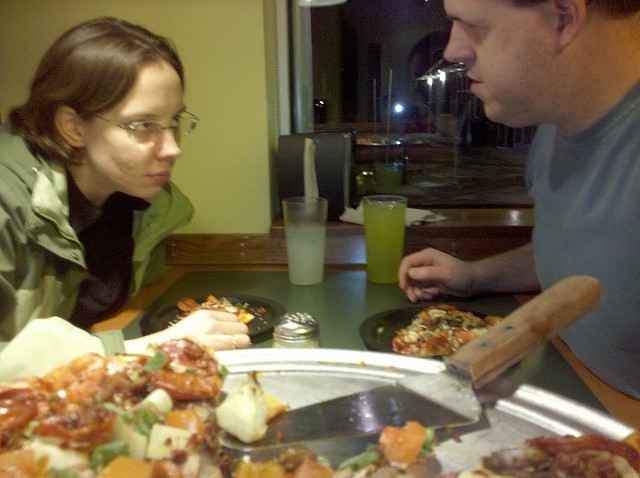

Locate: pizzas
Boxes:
[455,422,640,478]
[170,294,255,329]
[1,336,434,477]
[393,303,509,358]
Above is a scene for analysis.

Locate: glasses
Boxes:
[92,110,199,142]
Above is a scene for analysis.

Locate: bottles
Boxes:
[272,312,321,347]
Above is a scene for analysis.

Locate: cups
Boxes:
[282,196,328,286]
[362,195,408,284]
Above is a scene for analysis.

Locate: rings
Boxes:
[231,334,238,349]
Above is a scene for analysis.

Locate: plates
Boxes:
[359,307,518,369]
[140,294,286,335]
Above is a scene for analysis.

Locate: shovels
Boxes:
[215,275,604,452]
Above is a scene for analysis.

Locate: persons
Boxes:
[2,16,252,354]
[397,0,639,402]
[406,35,471,116]
